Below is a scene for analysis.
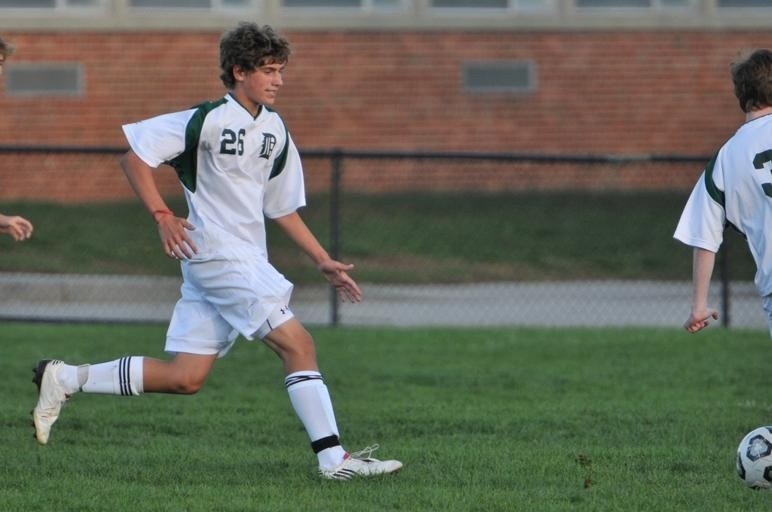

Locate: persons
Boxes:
[670,48,772,332]
[0,214,33,242]
[28,19,404,481]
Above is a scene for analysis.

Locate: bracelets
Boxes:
[152,208,176,225]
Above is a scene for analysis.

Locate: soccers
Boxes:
[735,424,772,489]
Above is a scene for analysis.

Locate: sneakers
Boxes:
[30,360,72,445]
[317,443,403,480]
